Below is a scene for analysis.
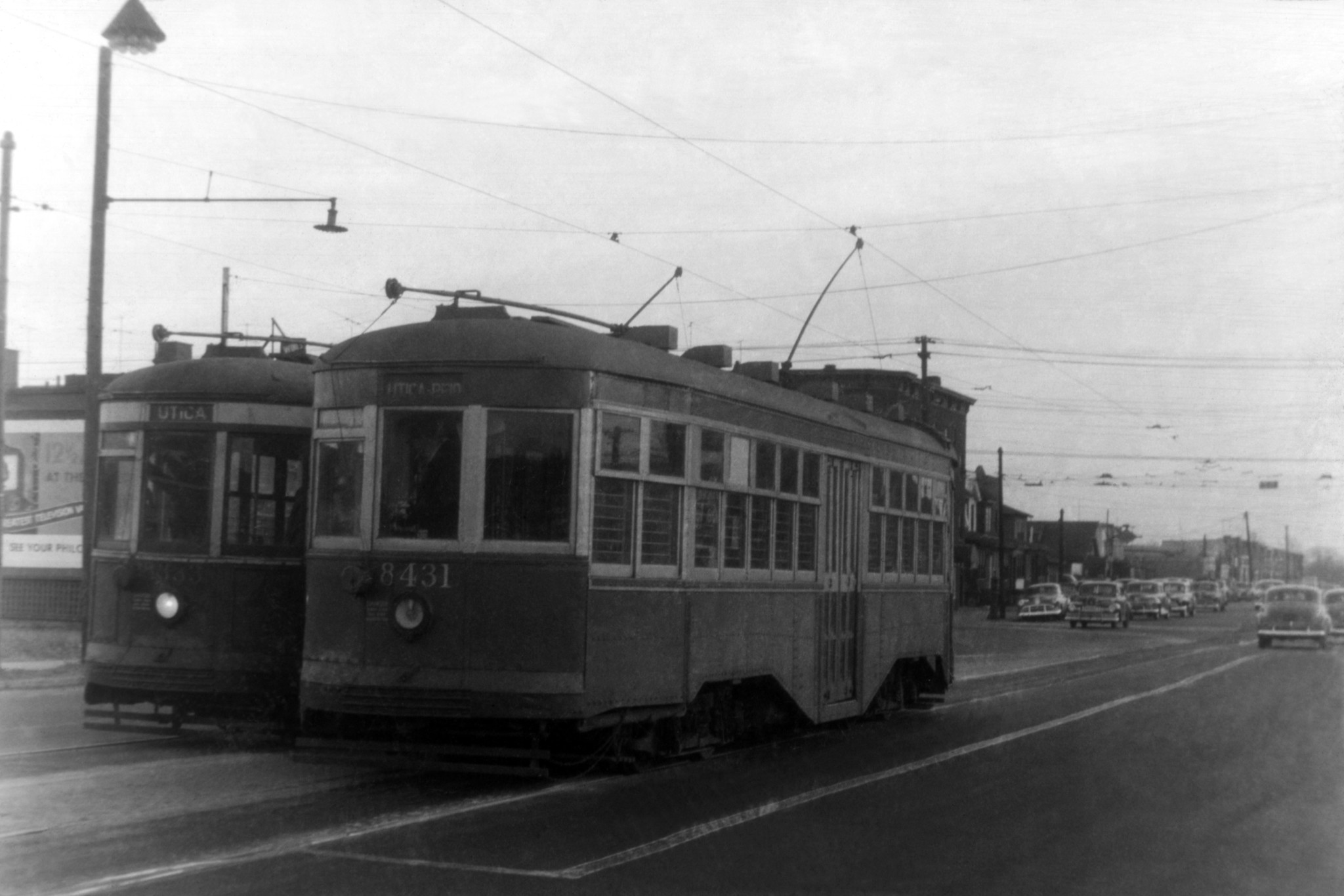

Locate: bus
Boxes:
[293,235,965,780]
[79,324,330,749]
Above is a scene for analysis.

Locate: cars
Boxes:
[1064,580,1129,629]
[1118,575,1344,650]
[1017,581,1071,622]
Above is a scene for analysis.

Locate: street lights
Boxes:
[74,0,171,669]
